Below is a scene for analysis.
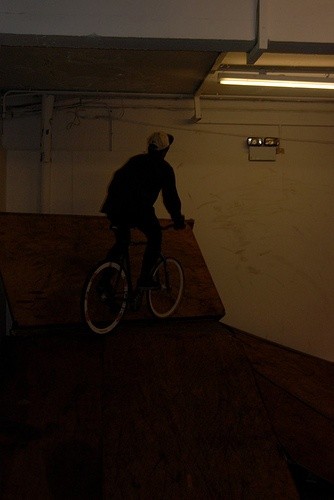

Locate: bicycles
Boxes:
[80,221,185,338]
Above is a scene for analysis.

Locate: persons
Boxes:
[99,132,187,288]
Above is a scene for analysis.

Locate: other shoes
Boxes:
[136,280,161,289]
[96,283,113,292]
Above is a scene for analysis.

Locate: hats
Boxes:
[146,132,174,150]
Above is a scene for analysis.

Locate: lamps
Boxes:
[247,137,280,161]
[218,73,334,90]
